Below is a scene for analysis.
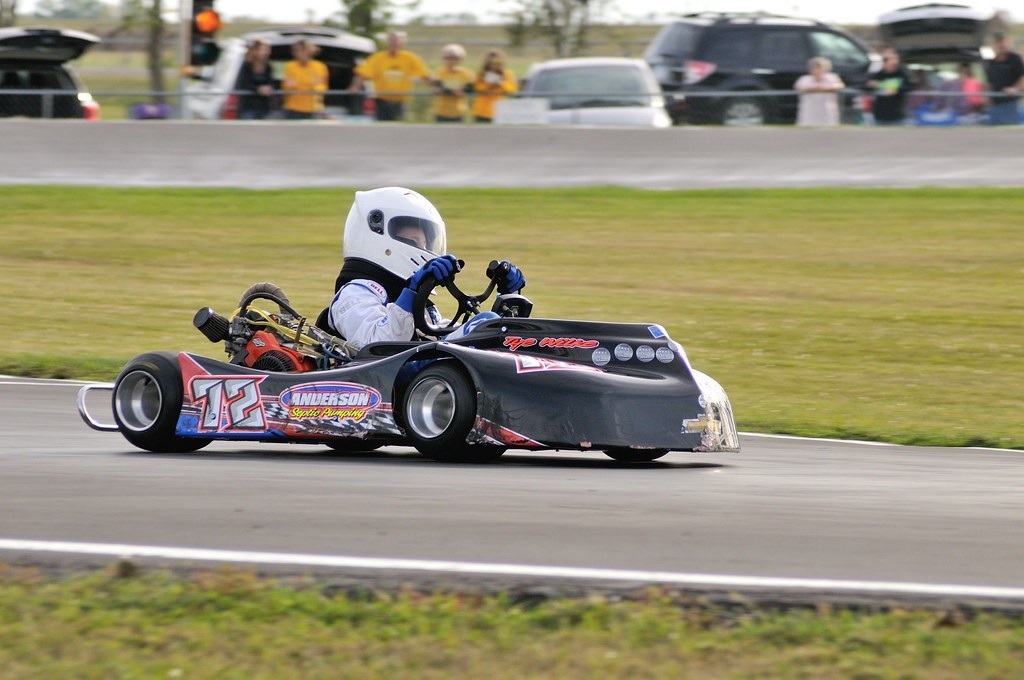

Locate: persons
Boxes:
[863,54,919,127]
[932,61,984,115]
[349,31,517,124]
[985,30,1024,125]
[282,39,329,119]
[236,40,281,120]
[793,56,845,125]
[316,187,525,352]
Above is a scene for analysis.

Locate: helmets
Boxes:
[344,186,448,295]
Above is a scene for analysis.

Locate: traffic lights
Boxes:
[190,0,221,66]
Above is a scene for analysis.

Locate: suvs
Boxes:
[645,9,918,125]
[185,26,384,124]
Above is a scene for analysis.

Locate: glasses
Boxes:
[488,59,501,64]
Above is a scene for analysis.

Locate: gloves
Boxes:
[486,260,525,294]
[395,255,461,311]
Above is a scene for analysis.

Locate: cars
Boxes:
[0,27,101,120]
[880,3,1004,126]
[486,55,674,128]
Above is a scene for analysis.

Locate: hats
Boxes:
[440,43,466,58]
